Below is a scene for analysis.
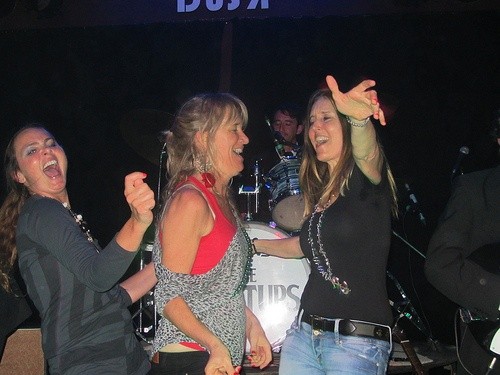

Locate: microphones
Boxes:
[404,183,426,225]
[265,119,286,158]
[453,146,469,174]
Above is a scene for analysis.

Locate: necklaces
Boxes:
[233,227,253,295]
[63,201,101,255]
[307,194,351,294]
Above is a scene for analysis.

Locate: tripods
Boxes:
[130,250,160,339]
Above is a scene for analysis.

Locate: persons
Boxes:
[251,75,398,375]
[0,123,157,375]
[425,113,500,375]
[263,102,303,170]
[150,93,274,375]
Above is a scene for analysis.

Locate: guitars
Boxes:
[481,325,500,358]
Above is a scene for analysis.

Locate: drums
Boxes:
[140,188,170,254]
[262,157,312,233]
[142,221,311,358]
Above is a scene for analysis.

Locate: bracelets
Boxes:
[252,238,258,254]
[346,116,370,126]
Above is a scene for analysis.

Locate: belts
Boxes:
[298,308,389,340]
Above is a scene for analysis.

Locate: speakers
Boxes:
[0,328,50,375]
